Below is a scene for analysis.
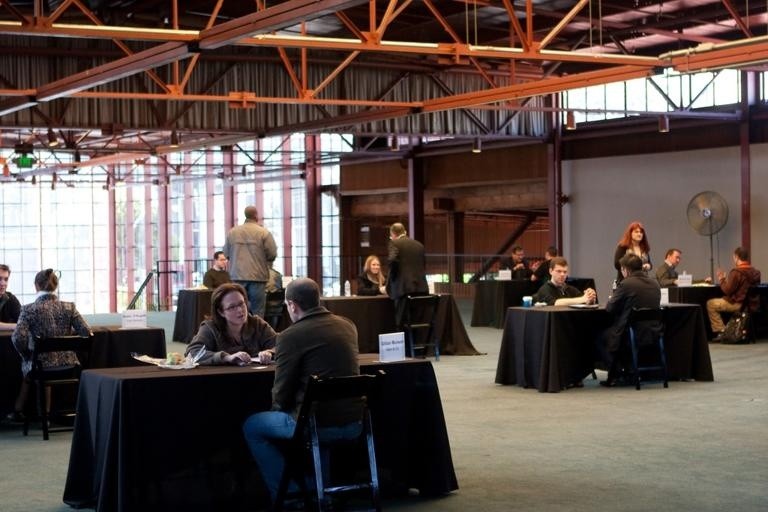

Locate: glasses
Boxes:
[220,301,249,309]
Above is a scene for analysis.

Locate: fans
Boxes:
[687,190,730,284]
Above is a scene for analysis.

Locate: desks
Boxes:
[5,321,165,407]
[181,288,241,327]
[668,285,768,324]
[495,301,713,389]
[55,359,460,509]
[284,292,490,360]
[469,278,600,325]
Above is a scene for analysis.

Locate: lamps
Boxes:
[392,136,400,151]
[567,111,576,129]
[170,133,179,144]
[46,127,59,147]
[472,137,480,152]
[659,116,669,132]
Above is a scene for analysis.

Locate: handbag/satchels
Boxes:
[722,306,749,342]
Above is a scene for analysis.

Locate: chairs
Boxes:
[608,304,672,389]
[736,283,768,342]
[8,336,90,437]
[273,371,380,512]
[385,295,443,362]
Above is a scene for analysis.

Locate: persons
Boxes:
[356,256,387,298]
[531,247,559,284]
[0,264,21,331]
[223,206,277,319]
[655,249,711,285]
[242,278,365,510]
[612,222,652,285]
[264,260,284,330]
[599,253,661,387]
[8,269,94,428]
[201,250,230,288]
[705,247,761,342]
[499,247,529,278]
[385,223,430,336]
[533,257,597,309]
[185,282,278,366]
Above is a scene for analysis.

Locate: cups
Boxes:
[522,296,533,307]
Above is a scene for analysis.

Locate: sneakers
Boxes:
[603,376,617,387]
[0,413,28,425]
[624,376,640,385]
[37,417,51,428]
[712,335,724,341]
